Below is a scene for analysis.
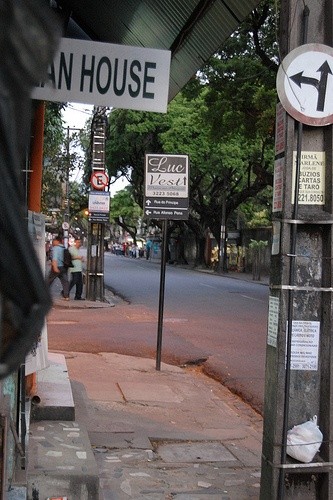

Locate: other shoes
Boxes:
[62,297,69,301]
[74,297,85,300]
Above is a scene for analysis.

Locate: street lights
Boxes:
[62,126,84,222]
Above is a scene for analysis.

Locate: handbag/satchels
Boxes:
[286,415,323,462]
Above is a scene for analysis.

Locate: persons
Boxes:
[60,239,86,301]
[46,237,69,300]
[45,241,50,261]
[121,239,152,261]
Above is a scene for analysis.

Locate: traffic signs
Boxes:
[144,154,190,220]
[88,191,110,223]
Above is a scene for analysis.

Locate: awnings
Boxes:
[55,0,262,102]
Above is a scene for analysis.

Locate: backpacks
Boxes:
[57,245,74,268]
